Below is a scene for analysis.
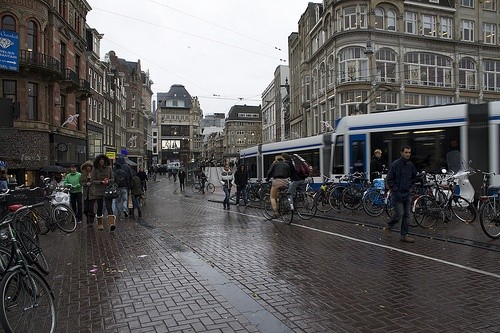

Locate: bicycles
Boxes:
[230,165,500,239]
[190,177,215,194]
[0,176,77,333]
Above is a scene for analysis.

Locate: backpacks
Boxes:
[289,154,312,177]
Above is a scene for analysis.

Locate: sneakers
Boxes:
[124,210,128,217]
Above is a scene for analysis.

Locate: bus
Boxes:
[240,80,500,206]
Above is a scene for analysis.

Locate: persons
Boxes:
[198,170,208,194]
[382,145,417,243]
[178,169,186,193]
[37,174,58,204]
[234,164,251,209]
[152,165,163,174]
[218,162,235,209]
[168,167,177,183]
[62,155,150,227]
[281,152,306,214]
[0,176,8,191]
[265,155,292,219]
[93,153,116,231]
[370,149,384,209]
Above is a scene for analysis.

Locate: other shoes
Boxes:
[223,203,226,208]
[227,207,230,209]
[237,204,239,206]
[119,218,122,220]
[244,204,250,209]
[400,233,414,242]
[138,211,142,217]
[383,225,391,236]
[290,205,294,211]
[71,219,82,223]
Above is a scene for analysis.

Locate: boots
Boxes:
[87,213,95,226]
[273,211,279,218]
[96,213,104,229]
[106,215,116,231]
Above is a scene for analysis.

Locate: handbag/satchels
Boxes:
[104,178,120,201]
[140,195,146,206]
